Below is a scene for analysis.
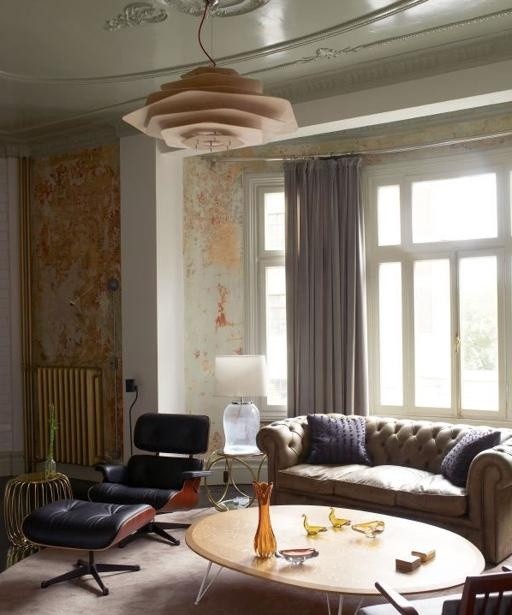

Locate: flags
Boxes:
[253,481,278,561]
[44,456,58,479]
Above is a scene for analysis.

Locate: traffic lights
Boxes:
[441,428,501,487]
[307,414,373,466]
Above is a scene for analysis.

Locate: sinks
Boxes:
[256,411,512,565]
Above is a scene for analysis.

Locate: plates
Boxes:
[204,451,276,511]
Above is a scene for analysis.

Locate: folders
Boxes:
[28,365,107,467]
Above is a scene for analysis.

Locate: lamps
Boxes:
[356,566,512,615]
[86,410,212,551]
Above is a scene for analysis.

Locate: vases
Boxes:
[44,402,61,459]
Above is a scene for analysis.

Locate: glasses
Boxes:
[213,355,270,455]
[117,0,305,158]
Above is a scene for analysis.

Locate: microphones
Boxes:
[18,497,159,602]
